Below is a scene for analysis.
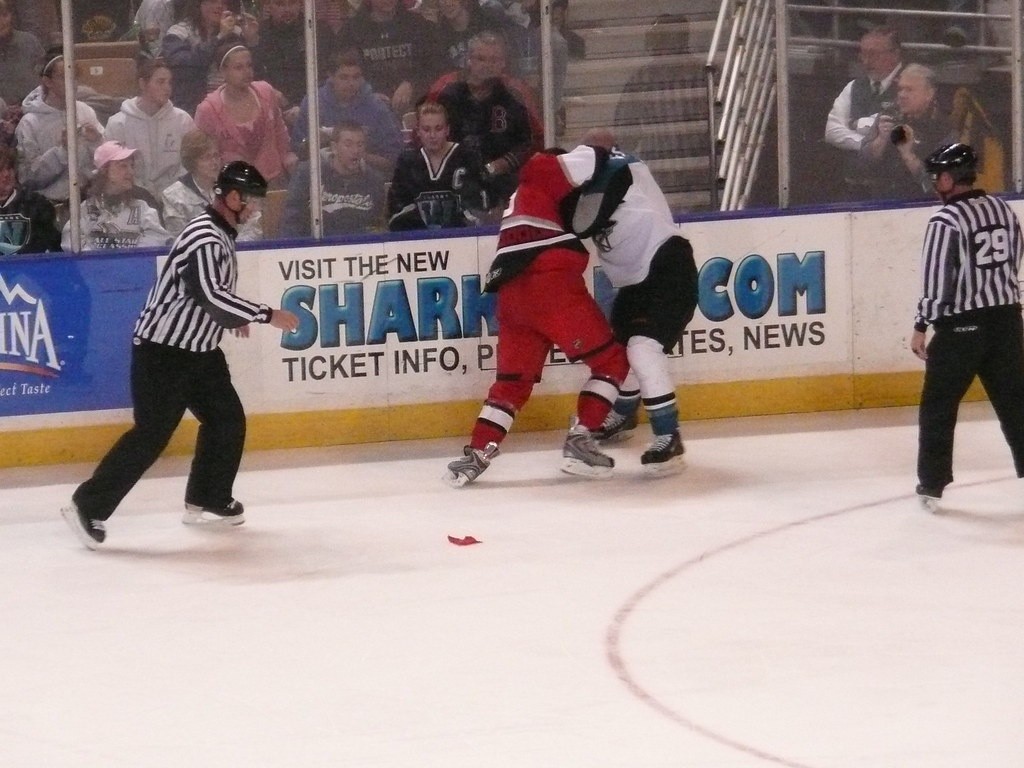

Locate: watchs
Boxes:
[486,162,497,174]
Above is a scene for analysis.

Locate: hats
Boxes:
[92,142,137,175]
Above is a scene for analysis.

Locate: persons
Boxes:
[257,0,336,106]
[910,142,1024,514]
[0,142,64,254]
[16,49,104,228]
[614,14,723,214]
[60,141,171,252]
[279,118,386,239]
[161,0,277,117]
[859,62,963,202]
[383,100,501,232]
[337,0,571,201]
[103,63,195,203]
[568,147,698,478]
[195,41,292,192]
[62,161,300,553]
[289,45,405,183]
[440,146,629,488]
[824,25,910,207]
[0,0,51,142]
[162,125,265,242]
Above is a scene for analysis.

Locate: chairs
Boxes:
[76,58,137,98]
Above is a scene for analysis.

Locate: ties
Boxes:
[872,81,882,95]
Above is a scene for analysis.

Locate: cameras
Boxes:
[233,14,245,25]
[884,115,906,146]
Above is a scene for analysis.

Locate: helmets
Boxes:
[216,162,267,197]
[925,143,978,179]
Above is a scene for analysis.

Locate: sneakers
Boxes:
[591,398,639,444]
[915,482,943,512]
[446,440,502,487]
[60,501,106,550]
[641,427,682,476]
[561,415,614,480]
[182,492,246,527]
